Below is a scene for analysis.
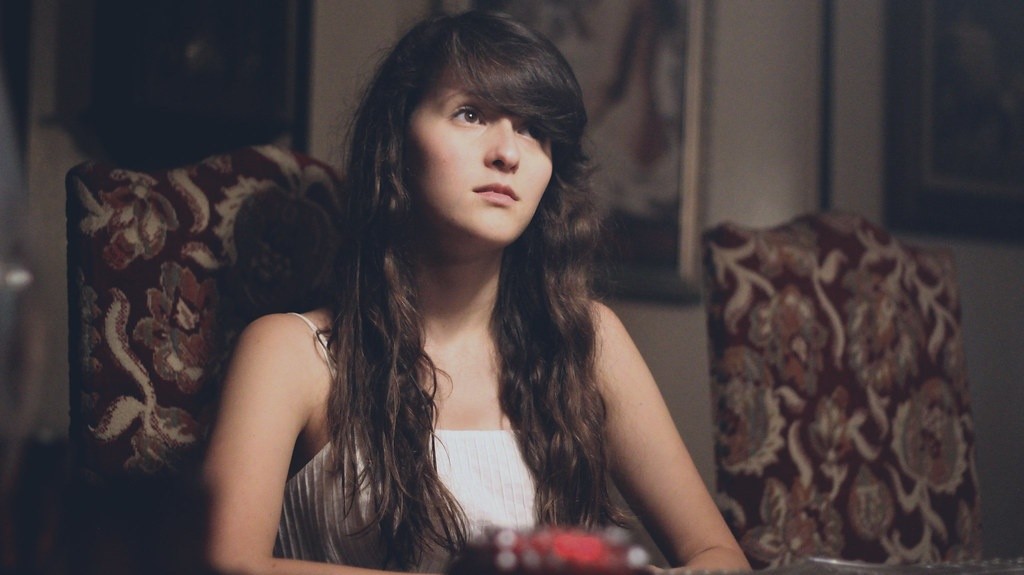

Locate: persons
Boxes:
[202,10,756,575]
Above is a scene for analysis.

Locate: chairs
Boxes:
[706,205,990,575]
[64,138,351,574]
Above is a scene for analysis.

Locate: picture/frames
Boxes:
[86,0,313,174]
[455,1,715,305]
[822,0,1024,269]
